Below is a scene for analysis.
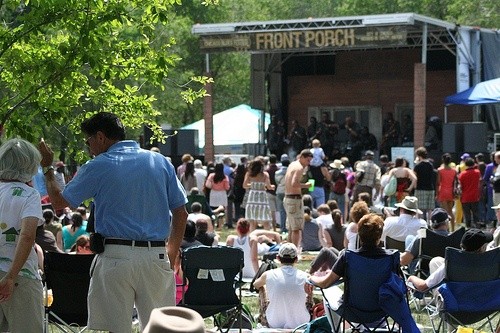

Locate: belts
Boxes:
[286,196,300,199]
[104,239,165,247]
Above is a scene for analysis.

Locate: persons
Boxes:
[227,218,260,278]
[400,208,452,274]
[309,246,339,278]
[0,138,45,333]
[56,168,65,186]
[486,203,500,249]
[310,212,393,327]
[35,206,92,254]
[267,111,378,168]
[402,114,414,147]
[310,139,325,167]
[37,112,189,333]
[253,242,313,328]
[165,243,190,304]
[407,229,494,333]
[382,113,400,158]
[177,149,500,251]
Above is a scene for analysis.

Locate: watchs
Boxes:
[43,165,53,175]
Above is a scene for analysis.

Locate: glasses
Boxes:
[84,136,93,146]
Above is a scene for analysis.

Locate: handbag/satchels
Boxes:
[216,304,255,328]
[292,315,332,333]
[206,174,213,188]
[453,178,462,197]
[333,179,345,193]
[384,169,397,195]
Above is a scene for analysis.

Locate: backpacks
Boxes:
[250,262,277,293]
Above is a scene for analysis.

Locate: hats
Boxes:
[329,159,345,169]
[394,196,424,214]
[461,229,493,252]
[461,153,470,159]
[278,242,298,259]
[142,306,206,333]
[430,208,448,225]
[491,203,500,209]
[182,154,194,161]
[475,153,485,160]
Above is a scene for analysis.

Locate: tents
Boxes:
[444,79,500,123]
[181,103,270,149]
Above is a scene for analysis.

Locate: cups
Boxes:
[308,179,315,191]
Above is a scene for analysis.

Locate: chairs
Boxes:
[401,227,466,313]
[304,250,409,333]
[180,246,245,333]
[185,195,225,228]
[405,247,500,333]
[41,253,96,333]
